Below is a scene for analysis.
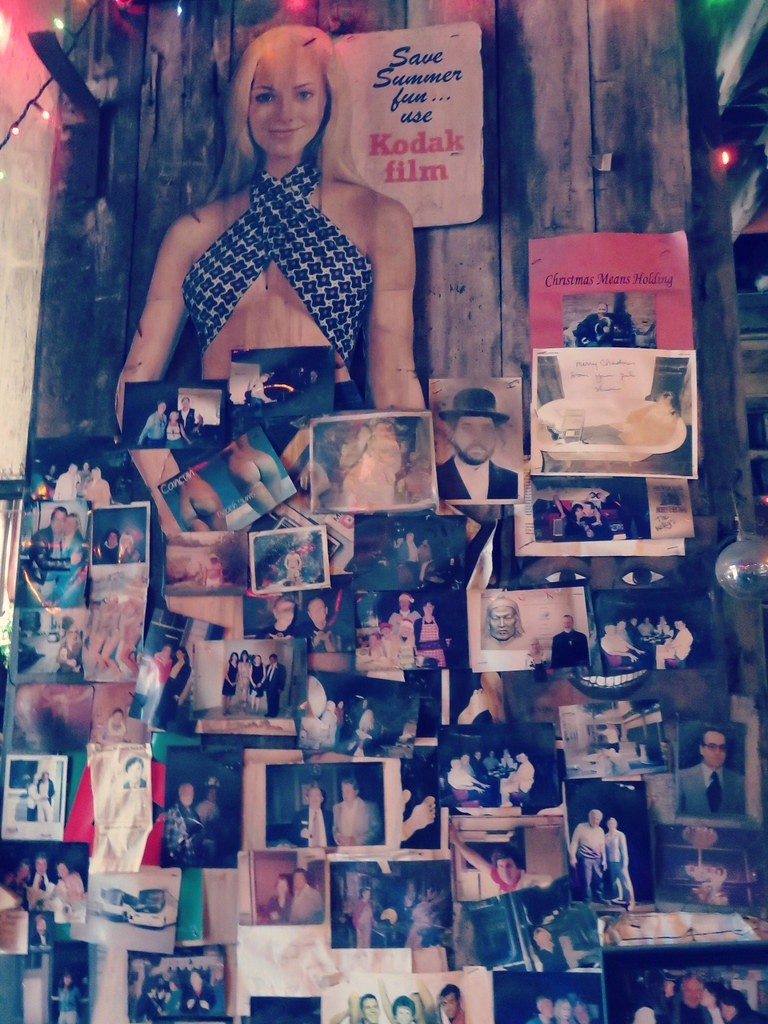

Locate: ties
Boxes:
[706,770,722,814]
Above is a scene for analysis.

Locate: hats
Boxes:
[438,386,511,428]
[357,876,373,894]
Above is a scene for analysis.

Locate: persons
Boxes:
[574,301,614,347]
[0,368,768,1024]
[113,25,431,535]
